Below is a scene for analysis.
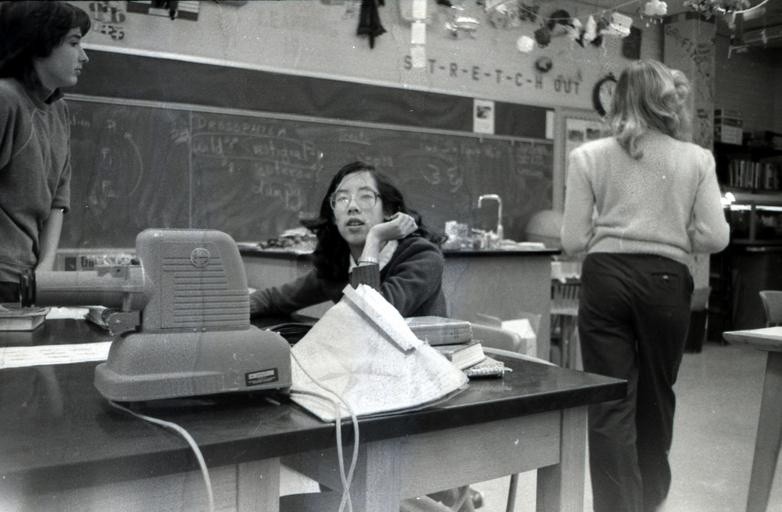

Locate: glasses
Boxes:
[330,189,382,210]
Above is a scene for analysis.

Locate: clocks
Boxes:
[593,73,617,117]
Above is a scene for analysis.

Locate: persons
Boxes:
[242,158,448,342]
[560,60,733,512]
[0,1,88,308]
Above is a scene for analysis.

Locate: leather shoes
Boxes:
[426,485,483,509]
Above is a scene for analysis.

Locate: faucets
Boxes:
[477,194,504,239]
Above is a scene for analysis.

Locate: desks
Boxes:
[722,326,782,512]
[0,319,629,512]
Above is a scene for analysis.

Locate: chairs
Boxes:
[471,320,521,353]
[759,290,782,328]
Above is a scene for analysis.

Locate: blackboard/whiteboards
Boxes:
[55,92,556,252]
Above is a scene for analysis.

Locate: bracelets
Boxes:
[356,255,380,266]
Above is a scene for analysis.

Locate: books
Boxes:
[465,359,508,382]
[729,159,777,188]
[400,313,474,348]
[428,341,490,372]
[0,303,48,332]
